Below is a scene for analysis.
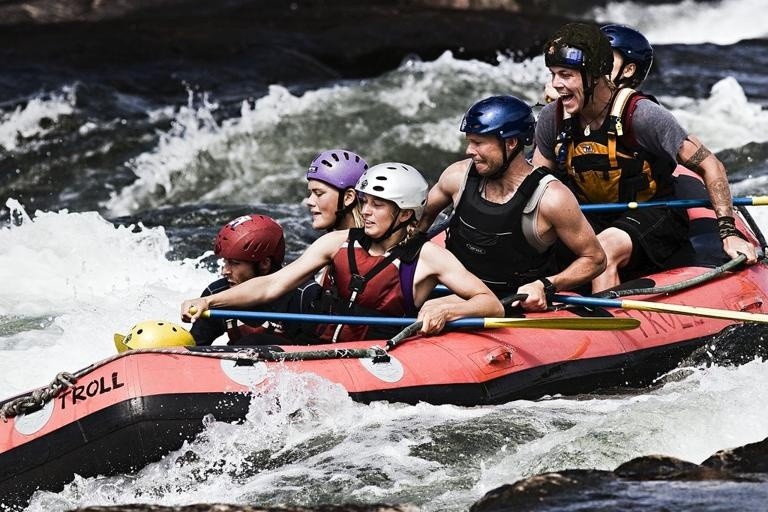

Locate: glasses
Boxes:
[545,42,589,66]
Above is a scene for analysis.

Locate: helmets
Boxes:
[459,95,536,146]
[601,23,653,90]
[215,215,285,267]
[544,23,613,77]
[307,150,366,191]
[355,163,427,223]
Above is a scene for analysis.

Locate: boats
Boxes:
[2,195,766,511]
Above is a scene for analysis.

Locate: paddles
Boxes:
[184,305,643,333]
[435,285,767,325]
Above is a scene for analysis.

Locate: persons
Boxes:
[529,22,759,296]
[181,147,505,345]
[412,94,607,313]
